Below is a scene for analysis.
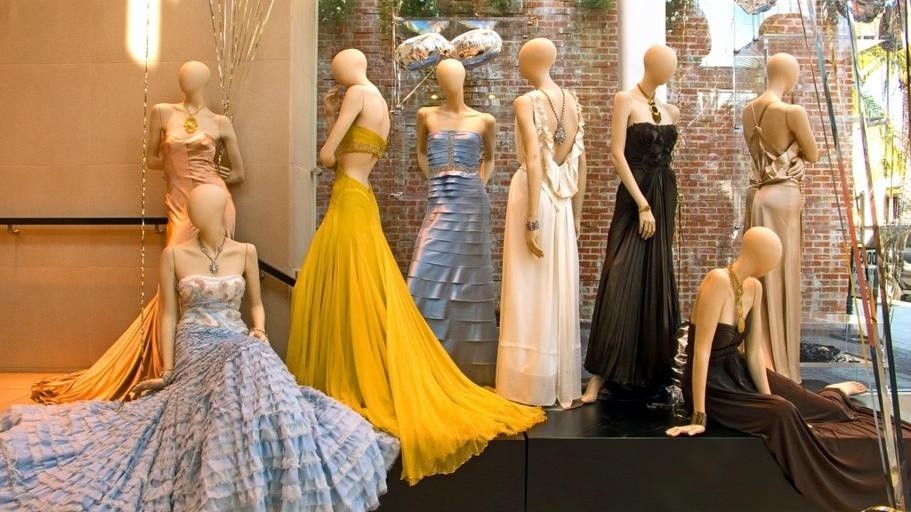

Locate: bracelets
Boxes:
[524,220,543,230]
[639,204,651,214]
[159,370,176,384]
[251,326,270,338]
[689,411,708,428]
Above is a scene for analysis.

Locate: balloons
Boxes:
[394,27,502,72]
[735,0,909,54]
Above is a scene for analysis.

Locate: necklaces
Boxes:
[534,84,568,150]
[197,231,228,275]
[636,82,668,129]
[176,94,211,131]
[725,264,750,338]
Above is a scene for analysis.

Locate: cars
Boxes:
[851,225,911,303]
[855,226,864,244]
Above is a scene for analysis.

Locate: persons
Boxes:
[31,55,245,407]
[284,44,548,485]
[664,224,871,438]
[502,37,586,412]
[741,54,819,382]
[1,183,400,511]
[579,41,687,406]
[410,58,500,386]
